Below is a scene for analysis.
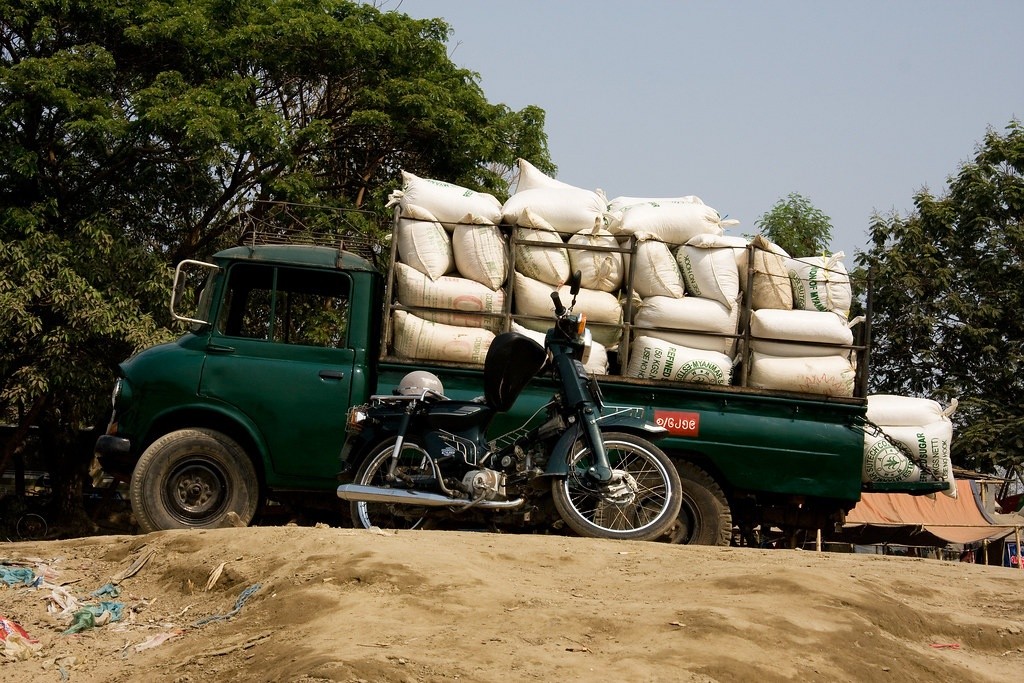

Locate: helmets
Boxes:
[397,371,444,402]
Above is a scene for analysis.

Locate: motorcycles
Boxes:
[337,269,683,541]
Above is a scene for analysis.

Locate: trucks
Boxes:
[89,203,875,546]
